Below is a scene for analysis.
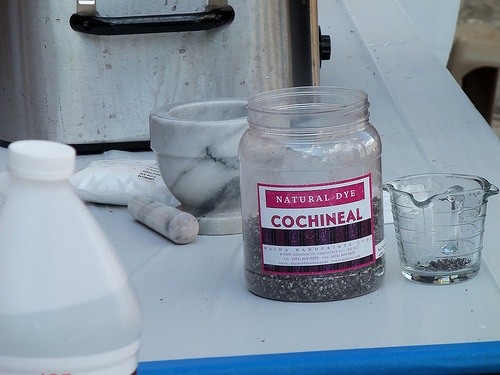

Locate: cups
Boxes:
[383,170,500,285]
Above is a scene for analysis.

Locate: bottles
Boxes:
[236,86,388,305]
[0,136,144,374]
